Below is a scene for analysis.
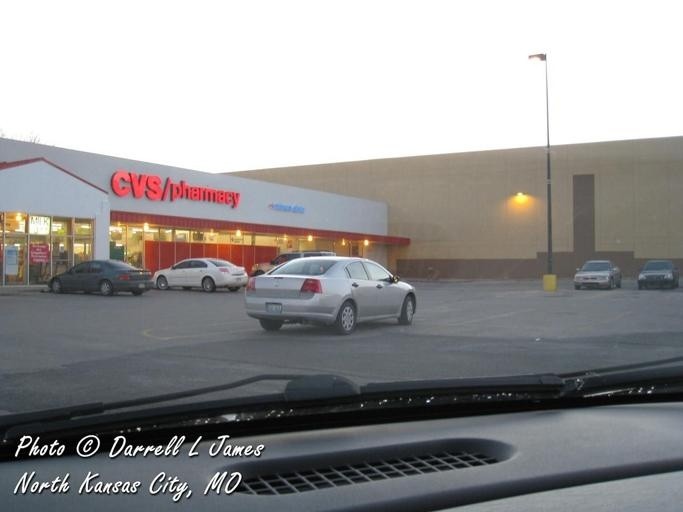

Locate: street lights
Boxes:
[530,53,558,292]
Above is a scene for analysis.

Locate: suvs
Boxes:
[638,260,680,291]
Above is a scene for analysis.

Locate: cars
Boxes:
[251,250,335,276]
[574,259,621,291]
[153,258,248,293]
[48,259,153,297]
[244,256,417,334]
